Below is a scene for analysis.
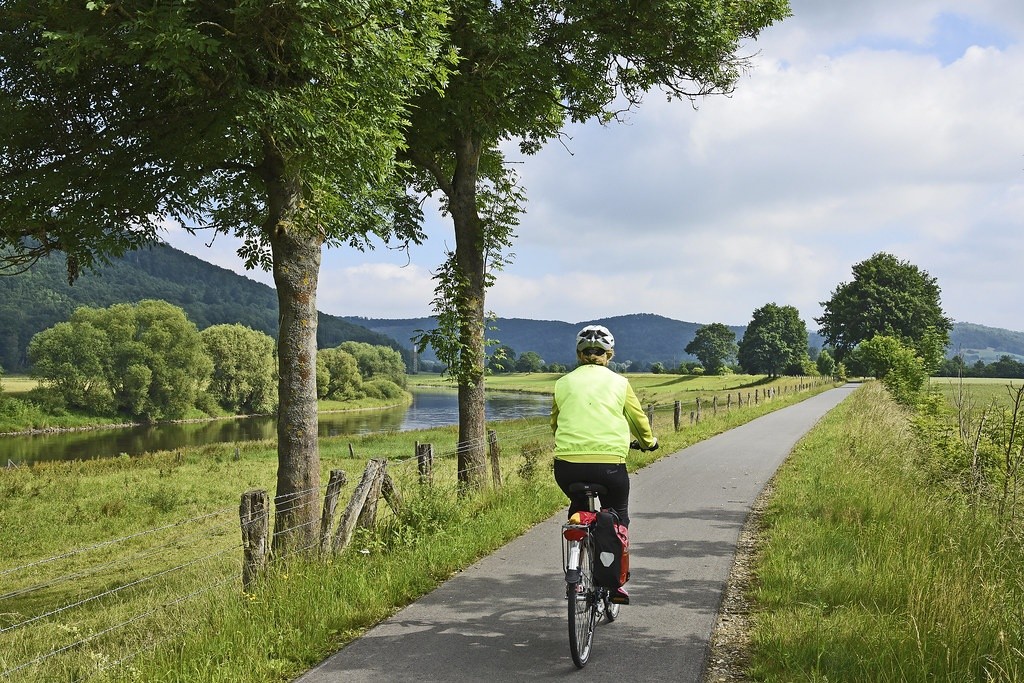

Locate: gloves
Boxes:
[643,438,658,450]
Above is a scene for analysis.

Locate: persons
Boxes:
[549,324,657,597]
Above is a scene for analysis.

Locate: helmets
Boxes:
[575,325,615,351]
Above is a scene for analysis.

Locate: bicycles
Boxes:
[557,439,658,669]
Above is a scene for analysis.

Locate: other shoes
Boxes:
[566,584,588,596]
[611,587,631,598]
